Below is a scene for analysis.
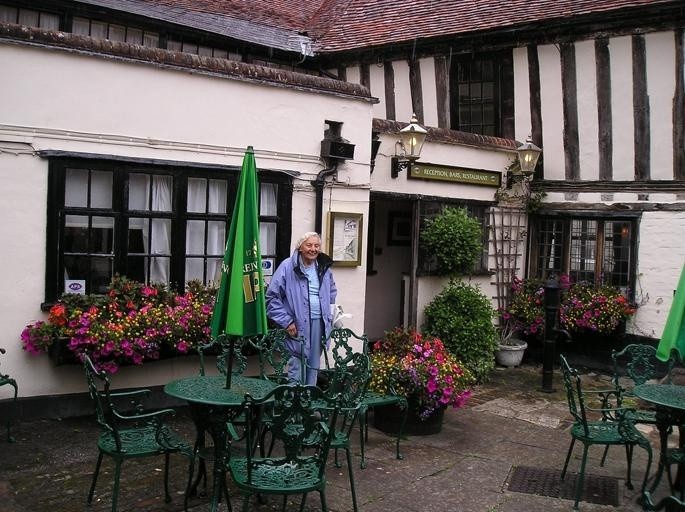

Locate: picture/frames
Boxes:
[327,211,363,267]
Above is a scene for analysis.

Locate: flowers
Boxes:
[19,274,218,372]
[555,274,638,334]
[495,275,547,345]
[368,324,478,422]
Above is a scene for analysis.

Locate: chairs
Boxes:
[600,342,678,466]
[78,352,193,512]
[558,354,652,510]
[0,348,18,442]
[642,492,685,512]
[216,382,343,512]
[197,325,408,470]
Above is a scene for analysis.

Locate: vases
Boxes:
[495,339,527,369]
[374,396,447,437]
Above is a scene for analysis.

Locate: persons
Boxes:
[265,231,338,386]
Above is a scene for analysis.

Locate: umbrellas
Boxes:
[655,262,685,364]
[211,147,268,389]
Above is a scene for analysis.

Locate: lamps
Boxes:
[506,134,542,189]
[391,111,428,178]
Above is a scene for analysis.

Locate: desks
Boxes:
[632,383,685,501]
[164,373,280,512]
